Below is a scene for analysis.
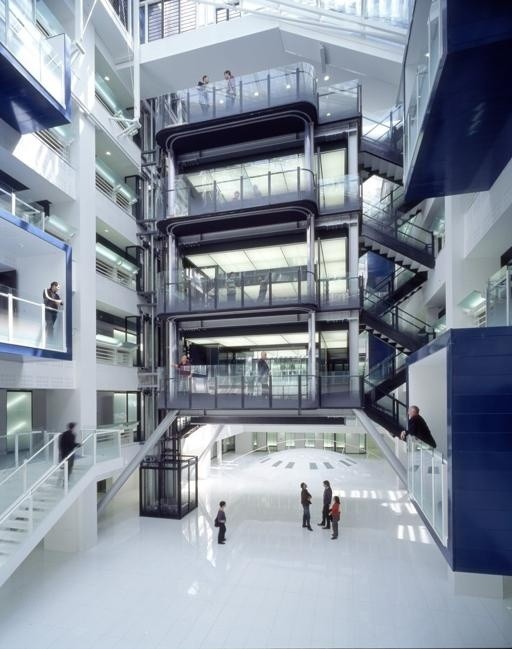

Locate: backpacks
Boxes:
[213,517,219,527]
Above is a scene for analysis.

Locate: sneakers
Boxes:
[218,538,227,544]
[317,522,331,529]
[331,533,339,539]
[301,524,314,533]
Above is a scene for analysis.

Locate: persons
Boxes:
[217,501,226,544]
[172,356,195,391]
[253,185,261,197]
[328,496,341,540]
[59,422,83,488]
[256,275,268,304]
[257,352,272,398]
[300,482,313,531]
[196,75,210,114]
[232,191,240,201]
[400,406,436,448]
[223,70,237,115]
[318,480,332,529]
[43,282,64,338]
[224,272,237,304]
